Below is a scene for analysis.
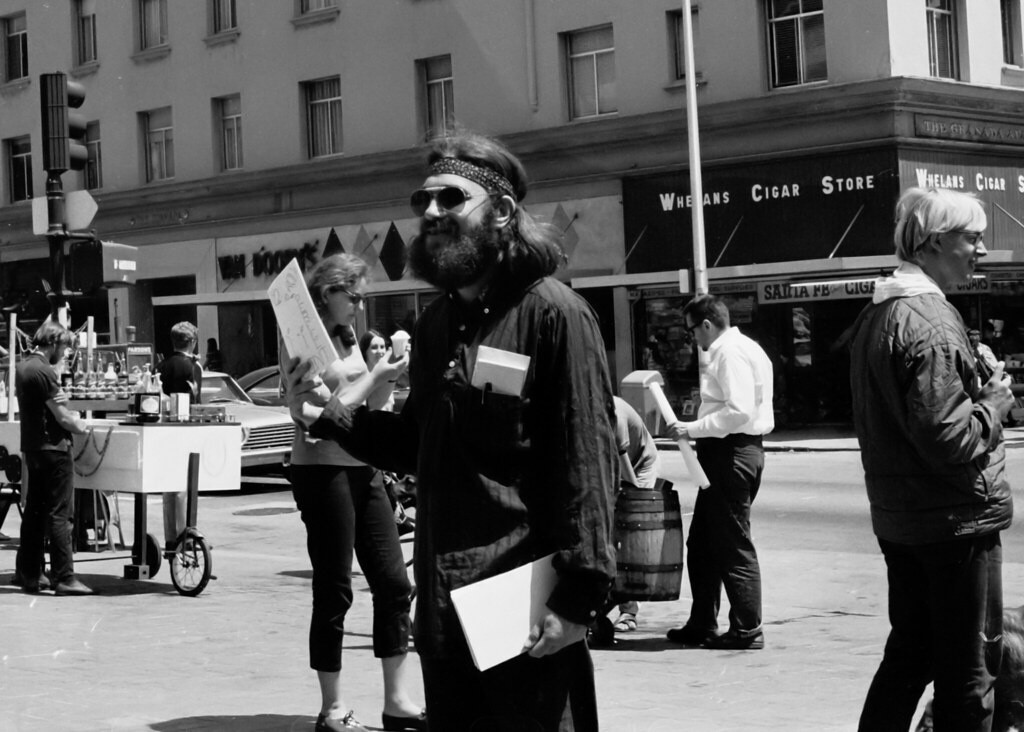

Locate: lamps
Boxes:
[678,258,695,293]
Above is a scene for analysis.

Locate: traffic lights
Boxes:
[38,68,90,170]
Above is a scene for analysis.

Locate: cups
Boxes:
[389,336,411,356]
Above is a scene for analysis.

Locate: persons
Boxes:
[612,394,663,631]
[204,338,225,373]
[152,322,204,553]
[843,186,1024,731]
[277,251,429,732]
[14,322,94,591]
[283,134,619,732]
[665,296,778,647]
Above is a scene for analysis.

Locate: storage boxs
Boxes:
[472,344,532,399]
[190,404,226,423]
[168,392,190,423]
[134,393,161,415]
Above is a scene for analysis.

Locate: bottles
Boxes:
[60,350,172,413]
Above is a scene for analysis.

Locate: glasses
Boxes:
[343,288,369,304]
[687,322,701,337]
[409,188,498,216]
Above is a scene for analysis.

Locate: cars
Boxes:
[192,371,297,468]
[232,364,412,417]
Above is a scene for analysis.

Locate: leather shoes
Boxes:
[25,582,56,593]
[56,583,100,596]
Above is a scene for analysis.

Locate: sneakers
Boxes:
[668,625,709,647]
[716,628,764,649]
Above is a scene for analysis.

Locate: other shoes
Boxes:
[315,710,378,732]
[382,708,426,731]
[614,615,637,632]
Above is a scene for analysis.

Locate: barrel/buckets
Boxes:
[609,488,684,601]
